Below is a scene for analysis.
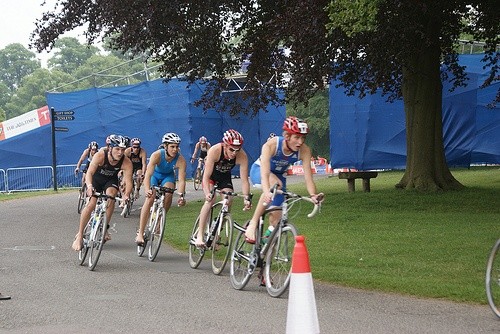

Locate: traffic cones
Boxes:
[285,235,321,334]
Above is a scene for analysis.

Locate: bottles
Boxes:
[260,226,274,246]
[92,217,98,227]
[151,211,156,223]
[209,217,217,232]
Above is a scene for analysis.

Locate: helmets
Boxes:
[131,138,141,145]
[124,136,131,145]
[88,141,98,150]
[223,129,244,148]
[283,116,310,136]
[199,137,207,143]
[162,133,181,144]
[106,134,127,148]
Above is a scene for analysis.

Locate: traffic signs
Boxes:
[54,116,76,120]
[55,126,69,132]
[53,110,75,115]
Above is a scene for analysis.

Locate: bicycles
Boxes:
[229,182,326,298]
[135,185,186,262]
[114,169,145,218]
[188,182,255,276]
[190,157,206,191]
[74,169,89,214]
[77,187,130,271]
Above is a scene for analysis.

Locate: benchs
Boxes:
[339,171,379,192]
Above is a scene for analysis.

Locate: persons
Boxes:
[195,129,253,251]
[190,135,211,184]
[71,135,133,251]
[243,116,324,286]
[74,136,147,208]
[136,132,186,245]
[311,154,327,166]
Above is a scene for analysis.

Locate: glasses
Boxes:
[228,147,239,153]
